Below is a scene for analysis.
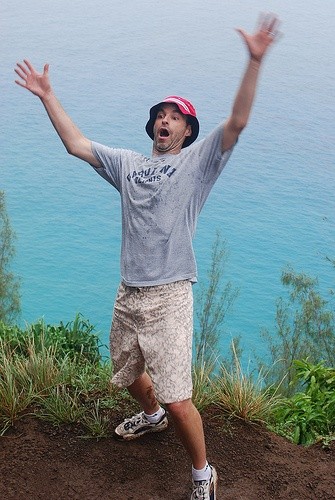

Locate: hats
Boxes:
[145,96,199,149]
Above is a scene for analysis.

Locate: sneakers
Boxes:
[190,465,217,500]
[113,408,168,441]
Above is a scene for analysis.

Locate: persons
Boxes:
[11,11,282,500]
